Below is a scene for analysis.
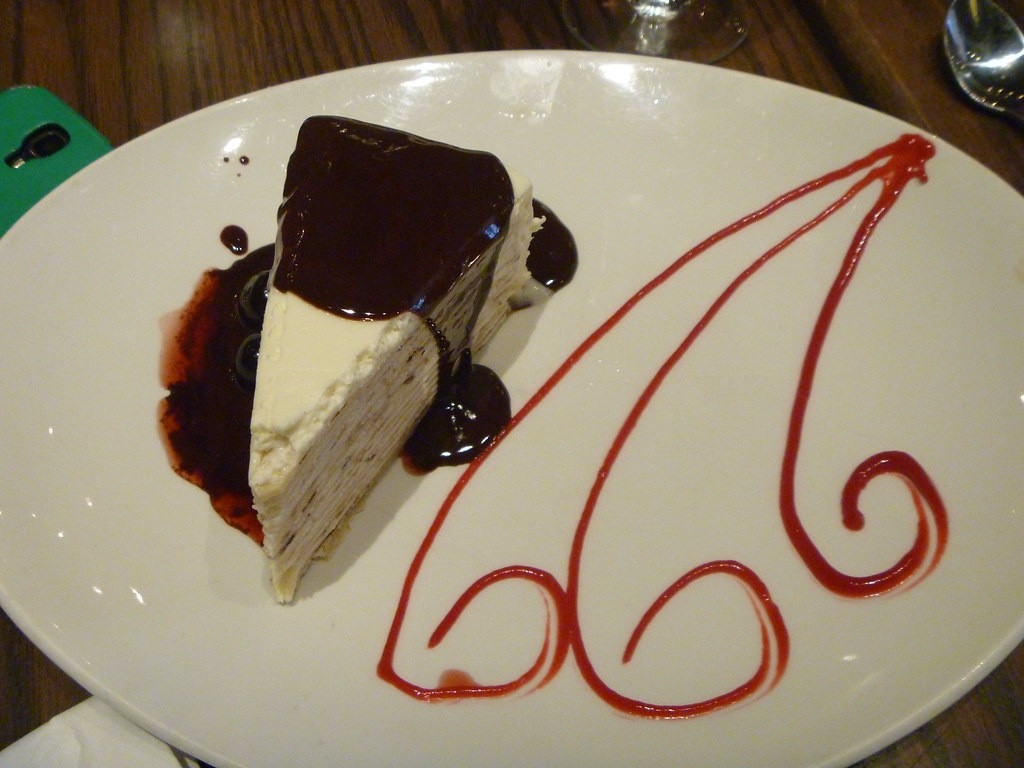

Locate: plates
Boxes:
[0,47,1024,768]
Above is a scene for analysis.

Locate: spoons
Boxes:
[943,0,1024,112]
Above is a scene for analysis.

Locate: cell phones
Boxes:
[0,84,114,240]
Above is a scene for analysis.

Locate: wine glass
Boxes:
[556,0,748,65]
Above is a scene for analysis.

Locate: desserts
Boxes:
[251,116,547,604]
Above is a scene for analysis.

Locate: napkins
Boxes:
[0,695,202,768]
